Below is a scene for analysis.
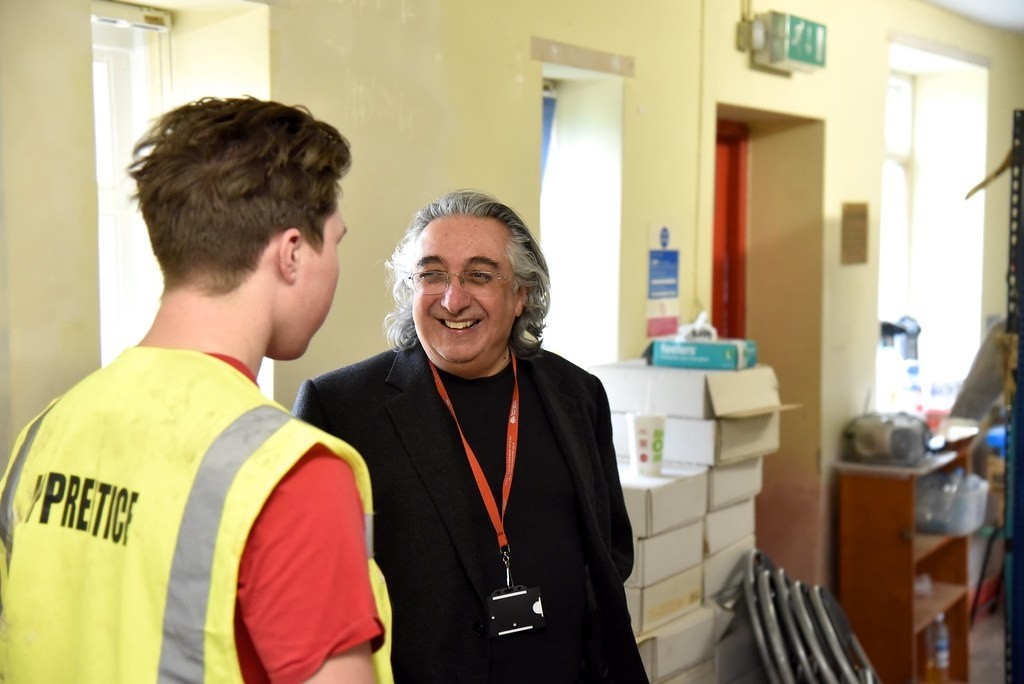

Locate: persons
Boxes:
[290,191,651,684]
[1,96,396,684]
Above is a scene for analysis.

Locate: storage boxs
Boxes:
[592,335,805,683]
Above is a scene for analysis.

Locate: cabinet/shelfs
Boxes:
[839,444,974,684]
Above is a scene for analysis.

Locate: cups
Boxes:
[626,413,668,476]
[924,409,951,450]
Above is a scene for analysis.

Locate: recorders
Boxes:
[849,413,932,466]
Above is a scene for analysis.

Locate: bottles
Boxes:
[933,612,950,670]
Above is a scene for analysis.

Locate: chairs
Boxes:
[744,550,882,684]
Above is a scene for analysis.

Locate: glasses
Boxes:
[409,271,517,295]
